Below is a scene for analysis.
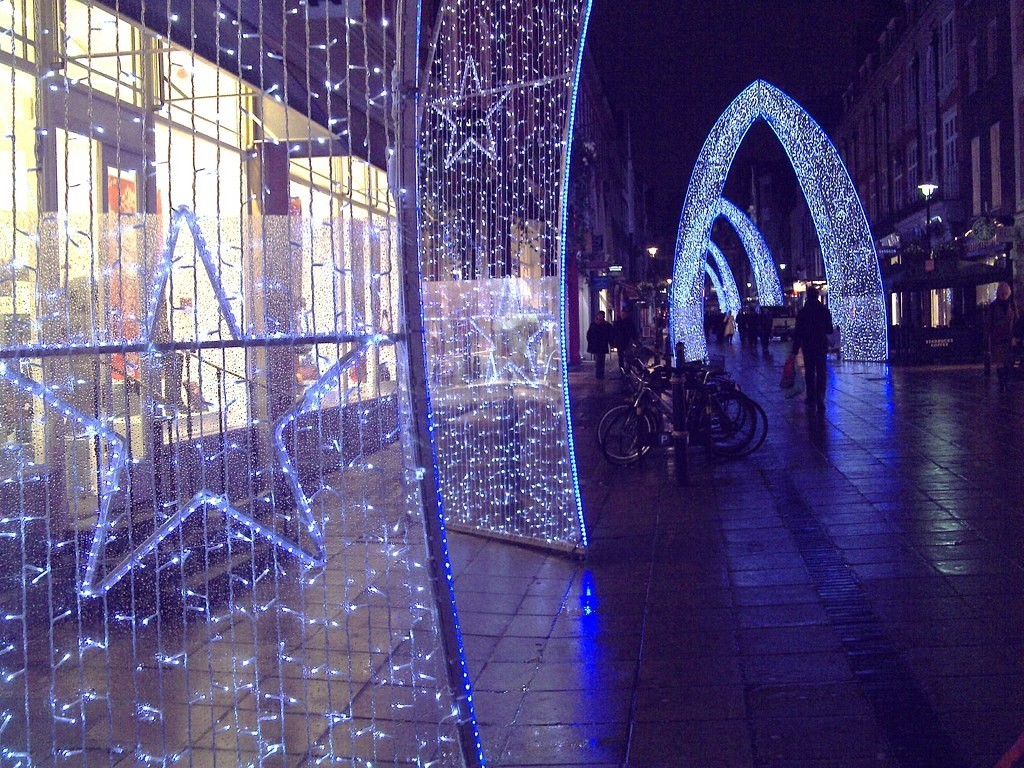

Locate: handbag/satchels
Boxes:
[781,353,796,388]
[784,358,805,399]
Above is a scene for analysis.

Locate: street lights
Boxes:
[919,182,937,329]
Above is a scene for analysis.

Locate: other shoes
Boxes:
[804,397,815,404]
[816,398,826,409]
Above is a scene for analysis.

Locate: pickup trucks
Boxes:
[763,305,796,337]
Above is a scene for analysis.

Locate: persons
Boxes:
[611,310,636,372]
[704,299,771,356]
[791,287,834,409]
[586,311,613,380]
[982,282,1019,393]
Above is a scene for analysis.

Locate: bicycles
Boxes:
[598,343,770,468]
